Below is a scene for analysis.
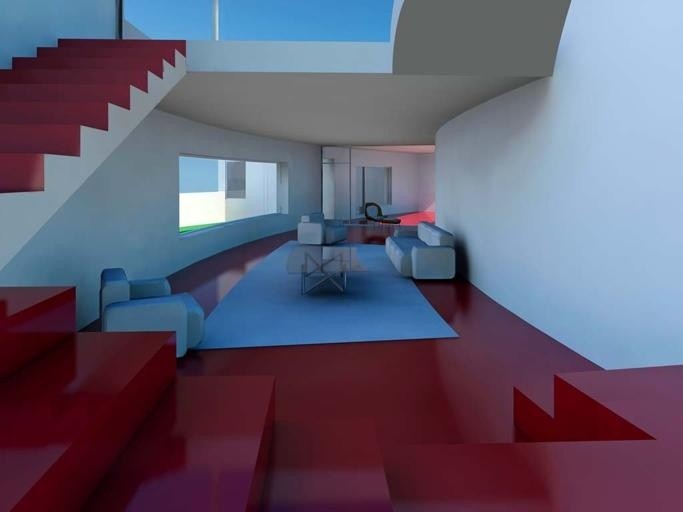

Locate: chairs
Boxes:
[365,199,401,242]
[98,268,204,360]
[298,212,348,244]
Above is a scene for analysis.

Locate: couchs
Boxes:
[386,219,457,286]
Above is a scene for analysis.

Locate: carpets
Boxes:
[187,239,462,352]
